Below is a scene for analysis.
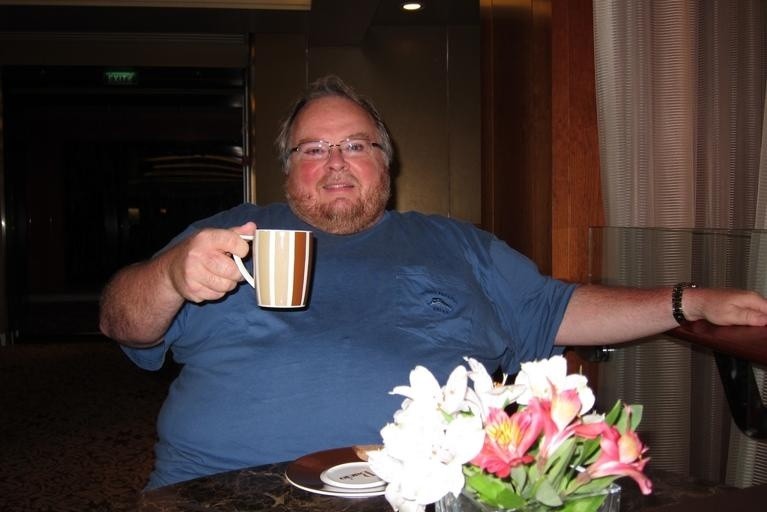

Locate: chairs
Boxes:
[503,317,766,445]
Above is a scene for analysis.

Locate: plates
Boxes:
[284,447,389,498]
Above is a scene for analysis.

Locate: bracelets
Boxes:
[671,280,698,327]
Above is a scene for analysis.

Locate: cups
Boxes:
[233,229,314,308]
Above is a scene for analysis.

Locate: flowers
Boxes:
[367,354,654,512]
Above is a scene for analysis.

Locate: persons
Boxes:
[99,76,766,495]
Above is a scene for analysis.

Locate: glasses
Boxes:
[286,136,385,164]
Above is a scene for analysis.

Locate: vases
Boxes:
[435,464,621,512]
